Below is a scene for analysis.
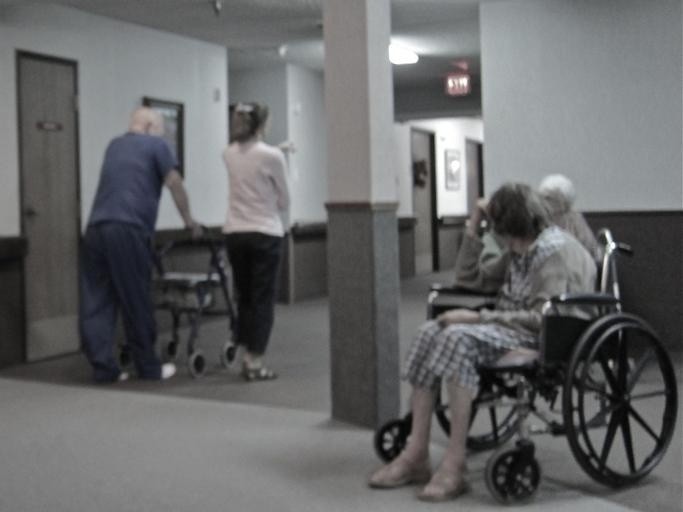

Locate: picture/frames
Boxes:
[141,96,184,180]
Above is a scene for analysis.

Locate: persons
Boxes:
[223,101,295,381]
[79,106,204,384]
[538,173,605,262]
[368,182,598,503]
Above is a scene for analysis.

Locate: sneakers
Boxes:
[117,362,177,382]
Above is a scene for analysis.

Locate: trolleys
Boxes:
[119,234,239,378]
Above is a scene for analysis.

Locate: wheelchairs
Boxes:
[374,228,678,505]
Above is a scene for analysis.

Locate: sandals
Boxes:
[367,448,470,503]
[241,359,279,382]
